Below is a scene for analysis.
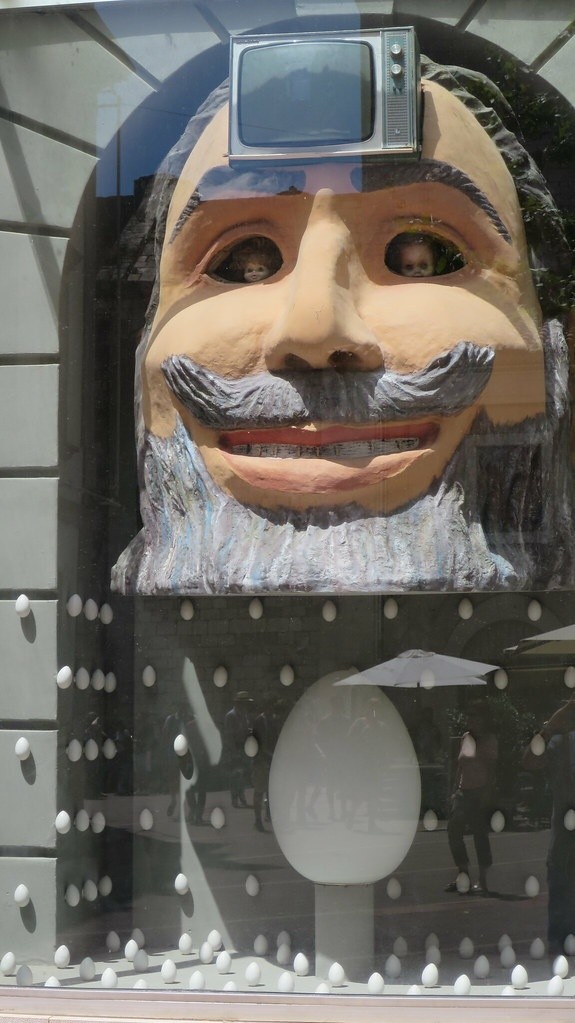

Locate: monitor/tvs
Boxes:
[227,25,420,170]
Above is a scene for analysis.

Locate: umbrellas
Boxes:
[504,624,575,692]
[332,647,500,772]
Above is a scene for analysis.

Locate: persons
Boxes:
[394,237,438,278]
[238,250,274,283]
[110,46,575,597]
[58,679,575,957]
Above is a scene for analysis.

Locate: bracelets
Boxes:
[543,721,555,733]
